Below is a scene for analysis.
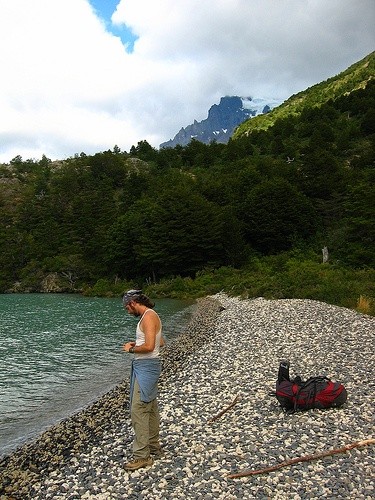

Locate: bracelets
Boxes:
[129,346,135,353]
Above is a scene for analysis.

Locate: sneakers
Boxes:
[124,457,153,471]
[150,453,161,460]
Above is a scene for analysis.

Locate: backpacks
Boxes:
[269,362,347,414]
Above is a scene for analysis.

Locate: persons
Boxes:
[123,290,165,470]
